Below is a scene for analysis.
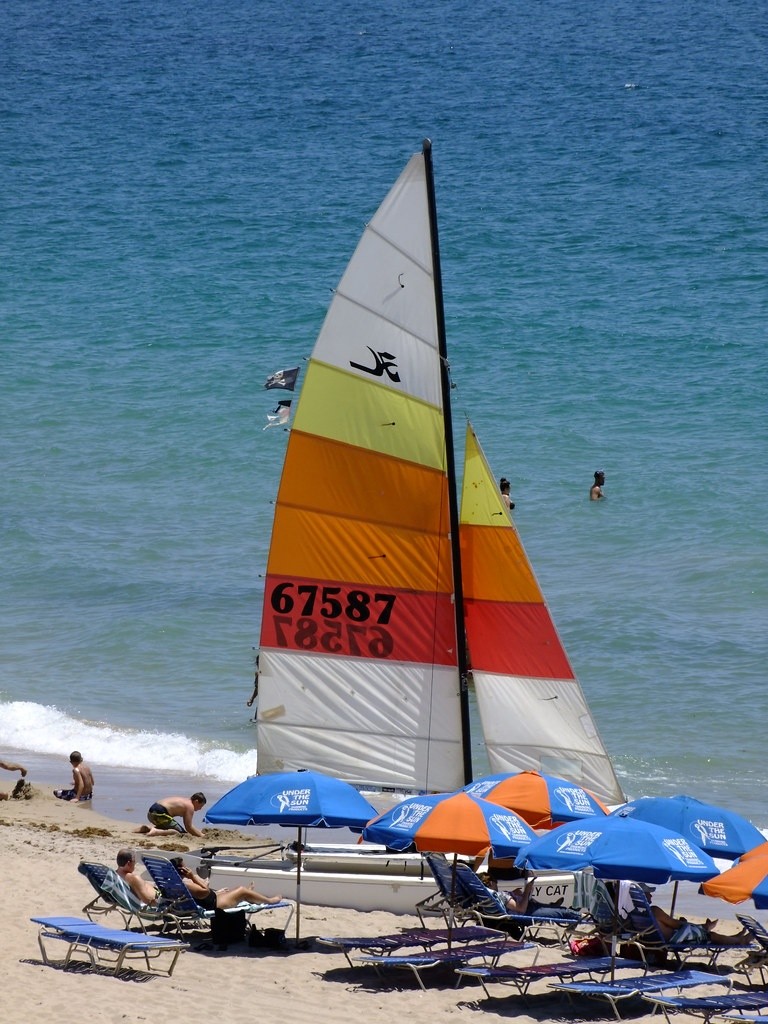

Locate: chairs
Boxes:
[29,853,295,978]
[314,851,768,1024]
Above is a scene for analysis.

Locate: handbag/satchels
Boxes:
[212,908,246,951]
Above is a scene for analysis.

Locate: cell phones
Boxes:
[177,867,186,875]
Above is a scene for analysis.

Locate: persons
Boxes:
[591,471,605,500]
[0,761,28,801]
[53,751,94,803]
[116,848,160,906]
[500,476,515,510]
[135,792,207,836]
[458,858,596,921]
[168,855,283,910]
[605,882,757,946]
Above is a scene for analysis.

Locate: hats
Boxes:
[636,883,656,892]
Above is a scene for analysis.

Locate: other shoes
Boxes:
[249,924,289,951]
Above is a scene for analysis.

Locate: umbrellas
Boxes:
[697,839,768,911]
[513,812,721,988]
[608,791,767,916]
[203,769,380,941]
[460,768,611,829]
[362,788,540,959]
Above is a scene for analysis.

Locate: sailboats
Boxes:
[130,142,627,915]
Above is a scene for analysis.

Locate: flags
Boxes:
[265,366,300,392]
